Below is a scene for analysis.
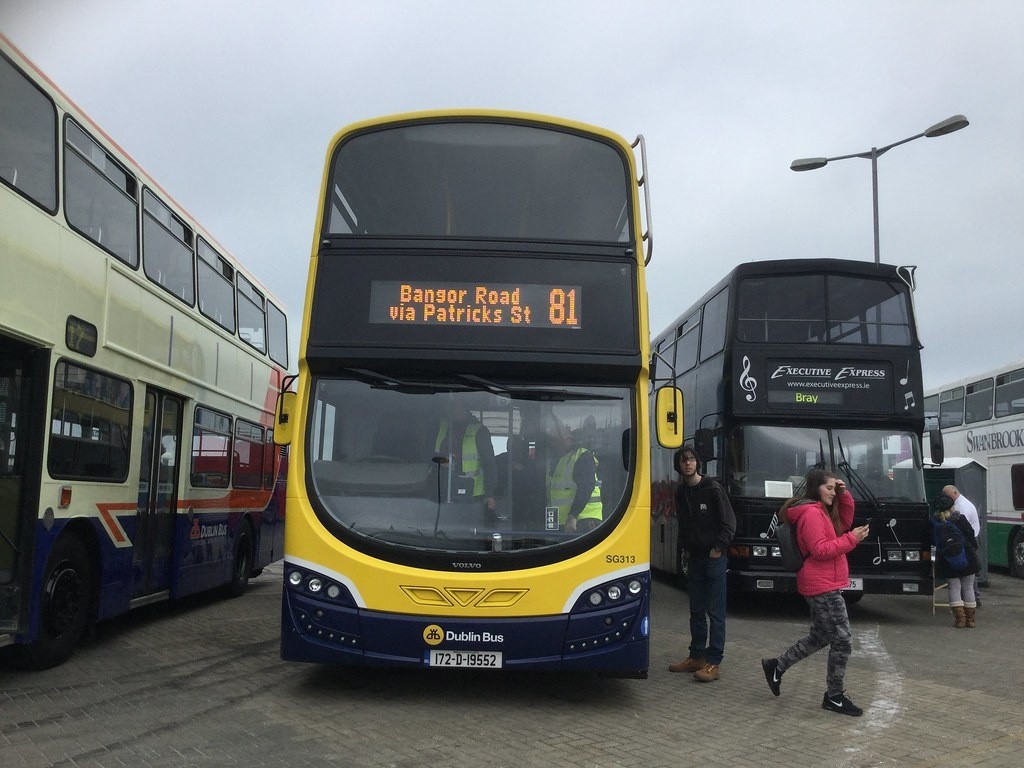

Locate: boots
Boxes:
[949,600,967,628]
[964,601,976,628]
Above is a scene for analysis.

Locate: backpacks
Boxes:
[775,522,810,573]
[931,514,971,573]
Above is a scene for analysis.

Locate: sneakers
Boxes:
[762,658,785,696]
[669,657,706,672]
[693,662,720,681]
[822,689,863,716]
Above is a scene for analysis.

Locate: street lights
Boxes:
[789,113,970,343]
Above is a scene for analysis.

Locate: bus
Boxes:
[0,34,289,672]
[836,359,1024,580]
[650,257,947,605]
[272,107,689,681]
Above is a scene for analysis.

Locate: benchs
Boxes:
[190,449,241,488]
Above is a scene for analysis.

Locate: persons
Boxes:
[493,426,603,534]
[429,404,496,528]
[933,497,982,628]
[761,469,870,717]
[669,448,737,680]
[942,484,981,606]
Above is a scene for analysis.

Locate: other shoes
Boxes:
[976,599,981,606]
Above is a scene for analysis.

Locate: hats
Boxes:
[932,493,954,512]
